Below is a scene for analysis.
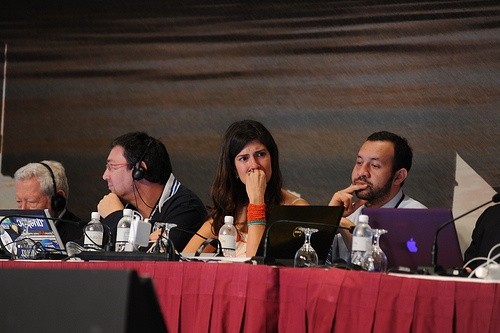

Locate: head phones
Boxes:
[154,225,219,254]
[41,163,66,212]
[132,138,153,181]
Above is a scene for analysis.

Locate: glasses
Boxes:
[106,162,127,170]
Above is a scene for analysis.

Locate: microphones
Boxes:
[0,214,88,227]
[257,218,355,264]
[418,194,500,275]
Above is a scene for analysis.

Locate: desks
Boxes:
[0,260,500,333]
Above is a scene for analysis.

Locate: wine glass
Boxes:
[294,227,319,268]
[156,222,177,253]
[360,229,388,273]
[12,220,35,260]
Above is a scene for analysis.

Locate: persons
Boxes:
[328,131,429,264]
[96,131,209,262]
[14,160,85,252]
[462,192,500,280]
[181,120,311,258]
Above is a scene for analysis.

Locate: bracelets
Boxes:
[246,203,267,225]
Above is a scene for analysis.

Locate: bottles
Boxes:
[114,209,133,252]
[350,215,372,265]
[83,212,103,252]
[217,216,237,257]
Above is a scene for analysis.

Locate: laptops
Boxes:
[256,205,345,267]
[363,208,463,275]
[0,208,67,259]
